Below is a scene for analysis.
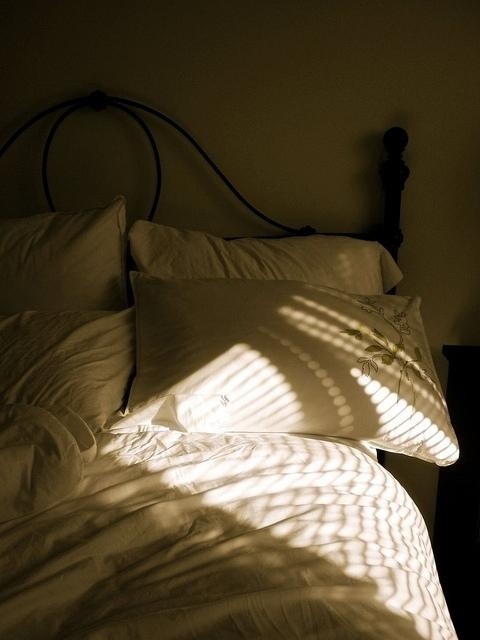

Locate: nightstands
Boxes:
[430,341,480,640]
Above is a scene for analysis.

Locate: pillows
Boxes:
[2,302,138,438]
[98,272,461,468]
[0,196,131,317]
[124,219,404,296]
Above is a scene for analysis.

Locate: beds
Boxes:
[1,82,462,639]
